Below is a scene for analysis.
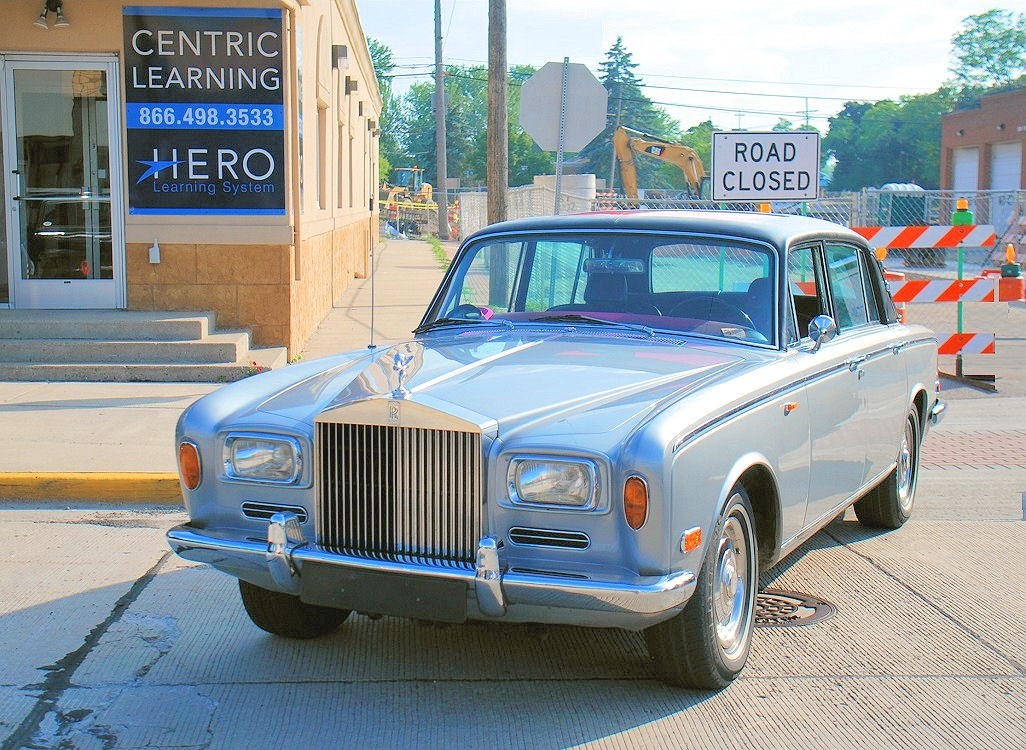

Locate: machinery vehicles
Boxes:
[378,165,438,239]
[613,125,757,211]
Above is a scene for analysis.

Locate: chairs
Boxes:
[545,273,627,312]
[743,278,812,338]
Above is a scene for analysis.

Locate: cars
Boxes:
[165,208,947,690]
[26,193,113,279]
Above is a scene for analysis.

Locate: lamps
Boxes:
[33,0,71,30]
